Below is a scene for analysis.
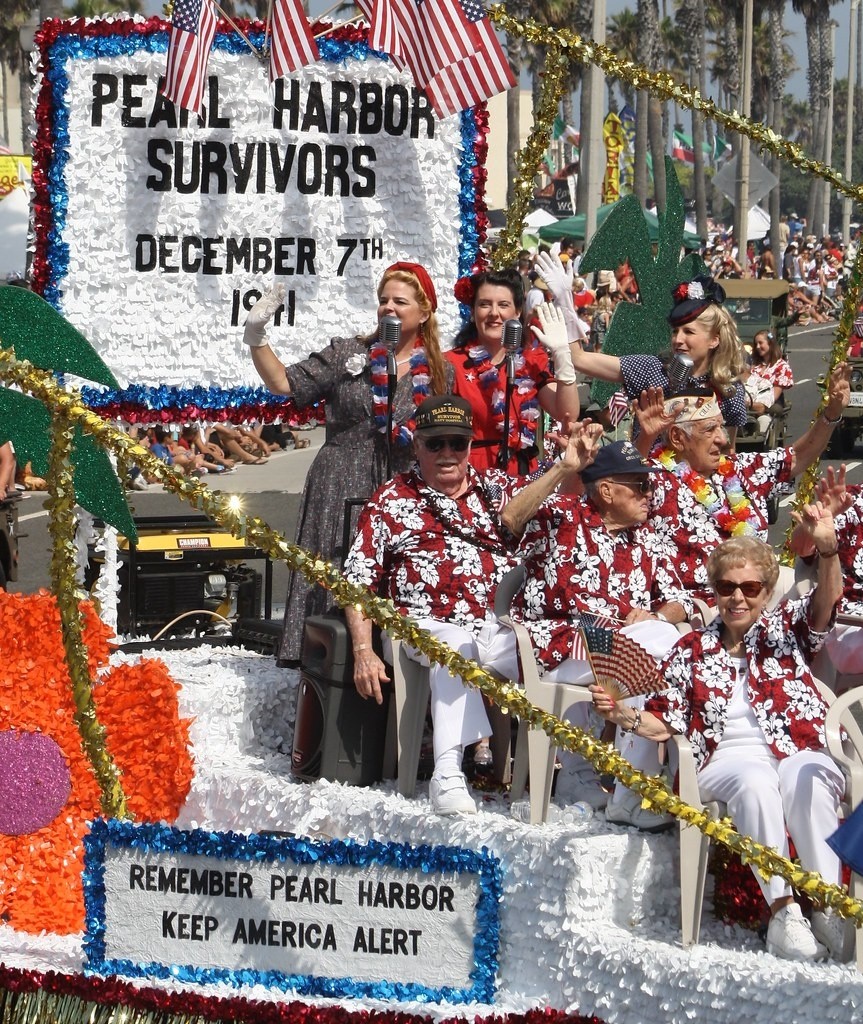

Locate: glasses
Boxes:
[604,479,655,494]
[714,578,767,598]
[418,436,470,452]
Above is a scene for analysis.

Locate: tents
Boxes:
[538,200,703,251]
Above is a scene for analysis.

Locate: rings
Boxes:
[592,702,596,708]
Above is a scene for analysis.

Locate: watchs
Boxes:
[652,612,668,623]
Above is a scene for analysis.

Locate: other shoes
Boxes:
[17,492,32,499]
[197,467,209,475]
[219,466,237,475]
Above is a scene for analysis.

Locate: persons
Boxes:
[0,200,863,963]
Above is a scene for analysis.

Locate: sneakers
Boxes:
[766,903,829,963]
[428,765,477,815]
[810,906,846,962]
[604,786,675,833]
[554,757,613,810]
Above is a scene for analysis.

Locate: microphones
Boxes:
[379,315,401,403]
[500,319,522,395]
[667,353,694,396]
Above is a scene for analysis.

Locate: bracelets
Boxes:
[352,643,372,652]
[816,540,840,558]
[820,406,843,427]
[621,706,641,732]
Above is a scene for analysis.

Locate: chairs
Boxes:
[383,559,863,979]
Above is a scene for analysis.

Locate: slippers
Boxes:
[243,456,269,465]
[250,448,263,458]
[297,438,310,449]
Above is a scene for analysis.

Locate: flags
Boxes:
[538,104,736,188]
[571,612,611,661]
[607,383,630,427]
[159,0,519,121]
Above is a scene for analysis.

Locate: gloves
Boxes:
[529,302,576,385]
[242,282,286,347]
[534,248,587,344]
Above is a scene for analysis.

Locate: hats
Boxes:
[797,280,808,288]
[411,395,473,438]
[580,439,661,483]
[791,212,799,219]
[648,375,721,422]
[558,253,573,262]
[737,349,753,366]
[596,276,610,287]
[721,259,733,266]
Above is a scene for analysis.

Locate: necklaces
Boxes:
[411,470,507,554]
[463,335,539,450]
[367,337,433,447]
